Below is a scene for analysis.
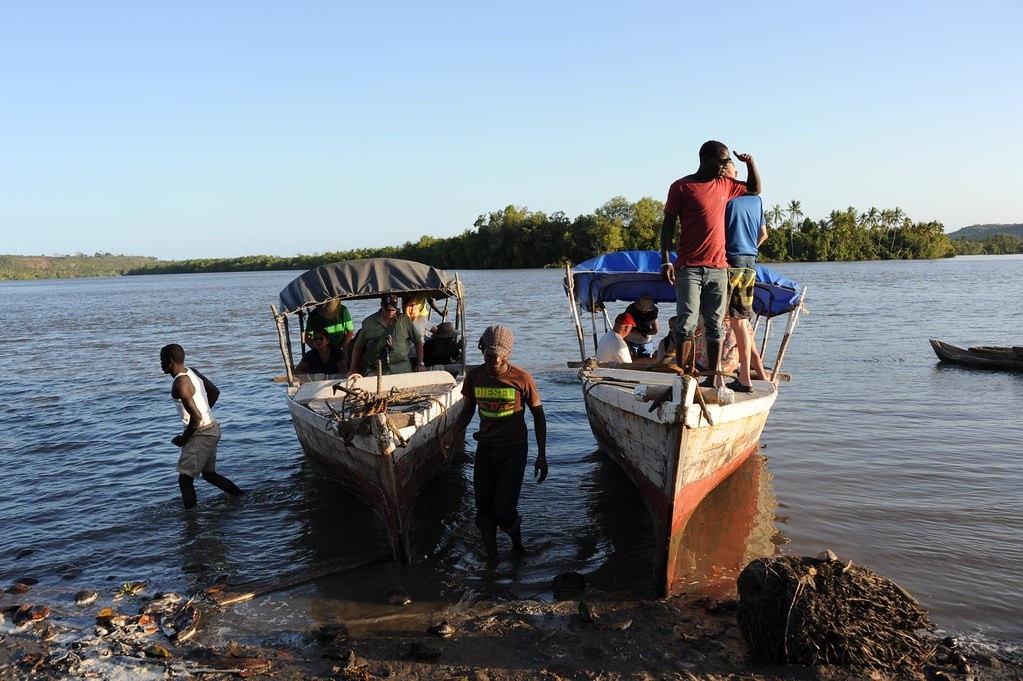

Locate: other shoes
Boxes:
[698,378,712,386]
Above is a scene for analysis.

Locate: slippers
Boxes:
[725,379,753,392]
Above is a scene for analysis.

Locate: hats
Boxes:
[478,326,513,355]
[381,293,399,308]
[635,295,655,311]
[316,298,341,318]
[431,322,459,338]
[615,313,636,327]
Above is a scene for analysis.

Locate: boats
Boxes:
[565,249,804,597]
[274,259,481,591]
[930,339,1023,374]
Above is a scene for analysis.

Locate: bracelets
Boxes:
[661,262,672,267]
[418,362,425,366]
[536,457,546,463]
[339,346,344,351]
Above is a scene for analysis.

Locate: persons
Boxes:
[660,140,769,393]
[657,316,676,364]
[698,159,754,393]
[305,301,354,354]
[294,329,347,374]
[160,344,243,509]
[624,291,658,359]
[423,321,462,379]
[596,313,637,363]
[402,297,437,370]
[694,313,776,390]
[440,325,548,556]
[345,294,427,378]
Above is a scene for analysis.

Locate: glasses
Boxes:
[712,158,732,165]
[313,337,324,340]
